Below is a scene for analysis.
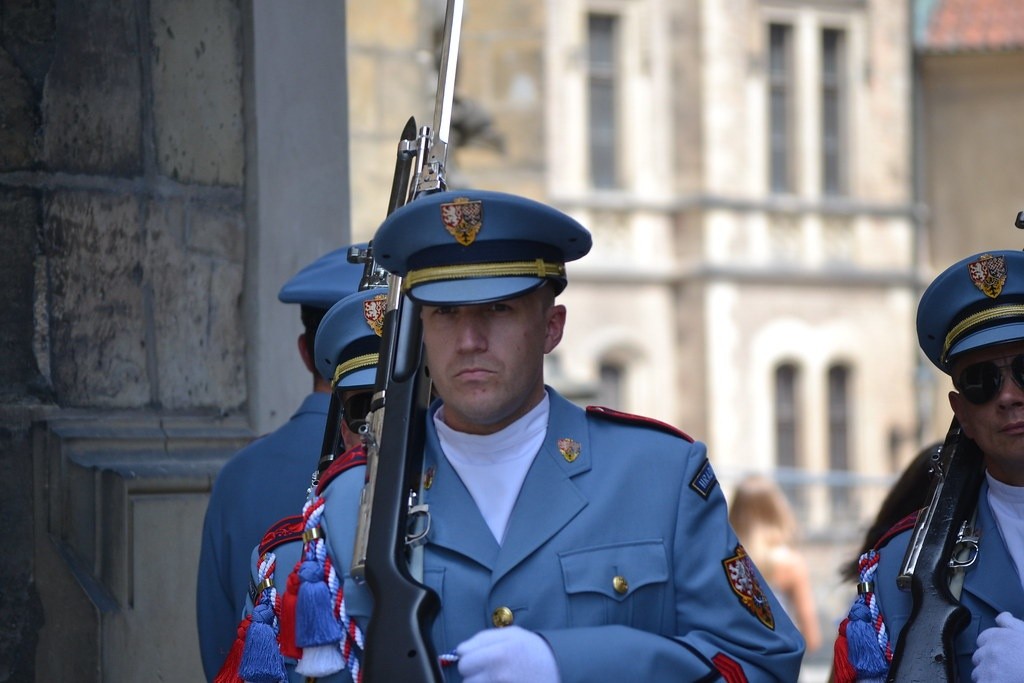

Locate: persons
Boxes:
[214,288,393,683]
[835,441,944,583]
[296,188,805,683]
[196,242,371,683]
[833,249,1024,683]
[728,473,821,654]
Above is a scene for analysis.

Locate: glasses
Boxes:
[340,390,374,436]
[958,353,1024,404]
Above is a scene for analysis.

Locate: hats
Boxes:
[278,242,379,329]
[372,188,594,306]
[313,287,390,398]
[916,249,1023,376]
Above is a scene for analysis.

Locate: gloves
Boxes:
[456,624,561,683]
[969,611,1024,683]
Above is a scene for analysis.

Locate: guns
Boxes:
[346,0,468,682]
[891,208,1024,682]
